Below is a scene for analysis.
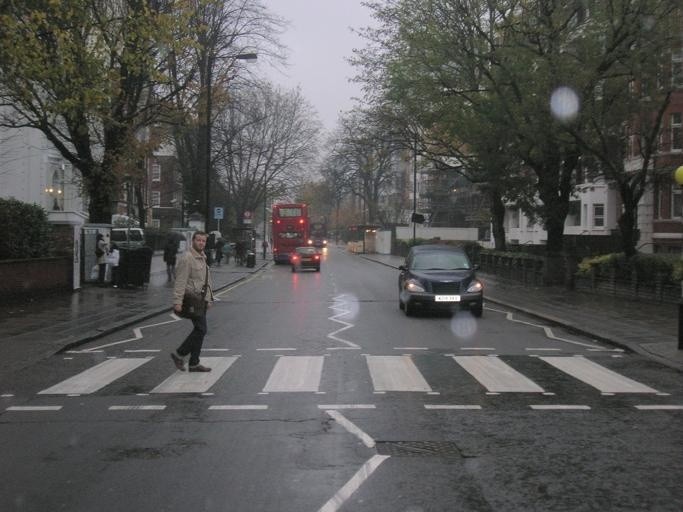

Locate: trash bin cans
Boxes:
[246,253,255,267]
[113,247,154,284]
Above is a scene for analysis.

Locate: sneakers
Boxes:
[189,365,211,372]
[171,353,186,371]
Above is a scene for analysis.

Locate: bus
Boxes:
[272,202,309,264]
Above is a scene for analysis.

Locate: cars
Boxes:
[397,243,484,319]
[290,247,320,273]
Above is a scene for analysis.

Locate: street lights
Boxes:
[203,53,258,264]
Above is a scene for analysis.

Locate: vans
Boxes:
[111,228,146,250]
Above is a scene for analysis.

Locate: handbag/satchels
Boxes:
[174,292,207,319]
[95,248,104,257]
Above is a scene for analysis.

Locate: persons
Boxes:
[97,235,122,290]
[172,233,215,372]
[162,233,268,282]
